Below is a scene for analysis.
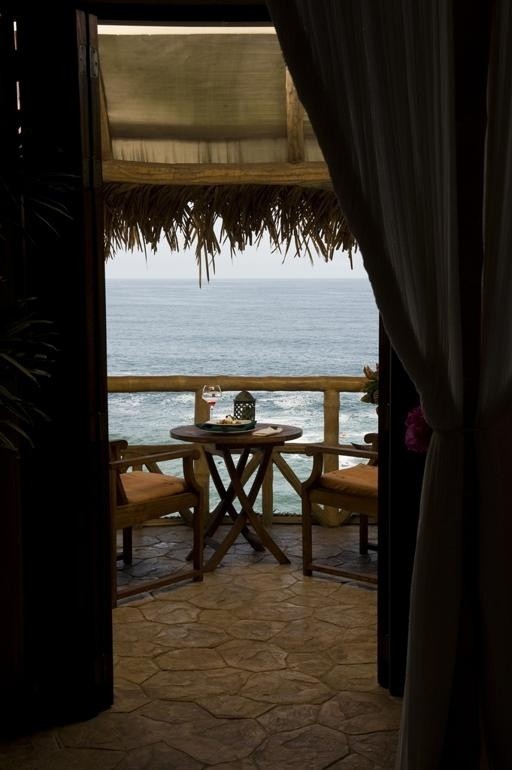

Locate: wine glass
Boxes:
[201,384,223,419]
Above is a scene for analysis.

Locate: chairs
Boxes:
[301,433,378,584]
[109,439,203,608]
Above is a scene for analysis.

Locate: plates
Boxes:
[204,418,252,427]
